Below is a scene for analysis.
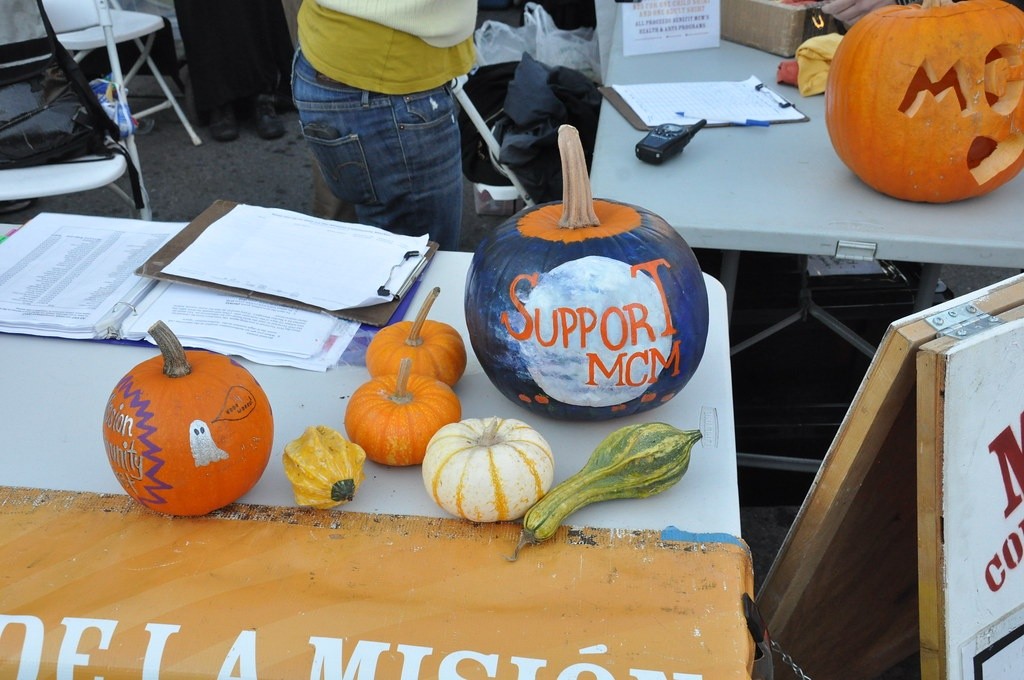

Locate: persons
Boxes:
[175,0,287,144]
[289,0,480,252]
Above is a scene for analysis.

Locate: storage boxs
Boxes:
[720,0,839,58]
[474,184,524,217]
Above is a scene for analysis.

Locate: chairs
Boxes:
[40,0,204,145]
[0,1,151,223]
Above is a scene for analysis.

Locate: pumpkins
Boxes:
[103,125,710,565]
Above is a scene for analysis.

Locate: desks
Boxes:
[591,0,1024,474]
[0,222,755,680]
